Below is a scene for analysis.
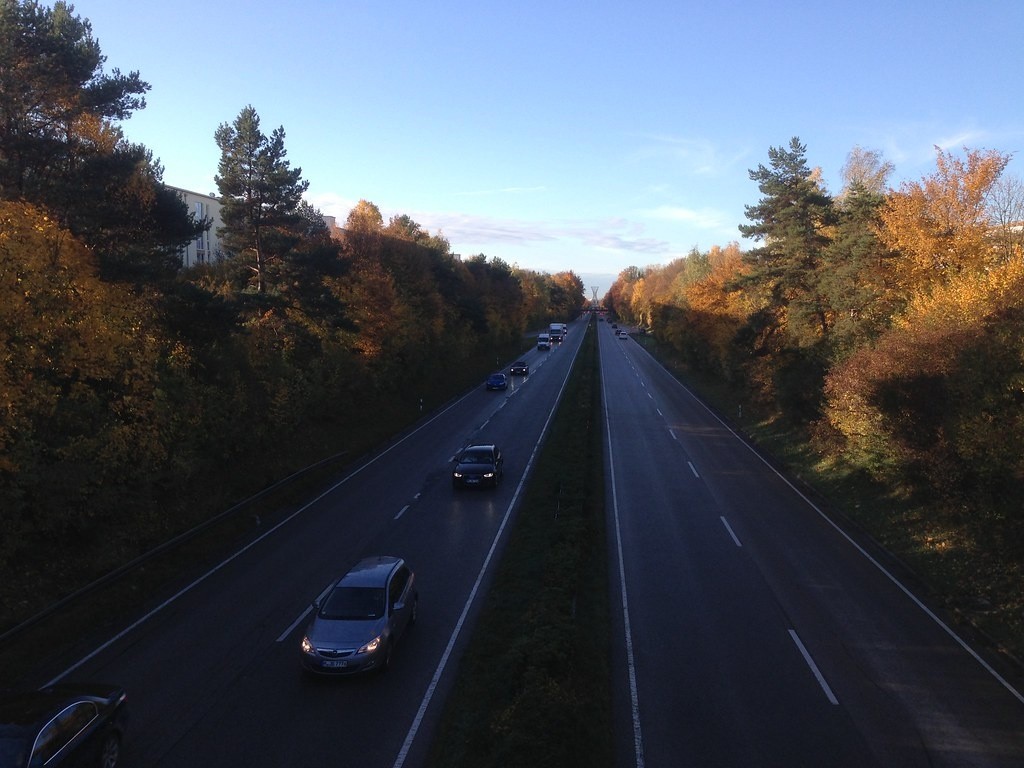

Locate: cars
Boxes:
[618,331,628,340]
[607,317,611,321]
[0,683,130,768]
[510,362,529,375]
[612,324,617,328]
[451,442,504,491]
[486,373,508,391]
[616,329,621,334]
[298,556,419,674]
[608,319,612,323]
[599,318,603,321]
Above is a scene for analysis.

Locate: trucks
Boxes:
[537,333,551,349]
[563,323,567,334]
[550,323,564,342]
